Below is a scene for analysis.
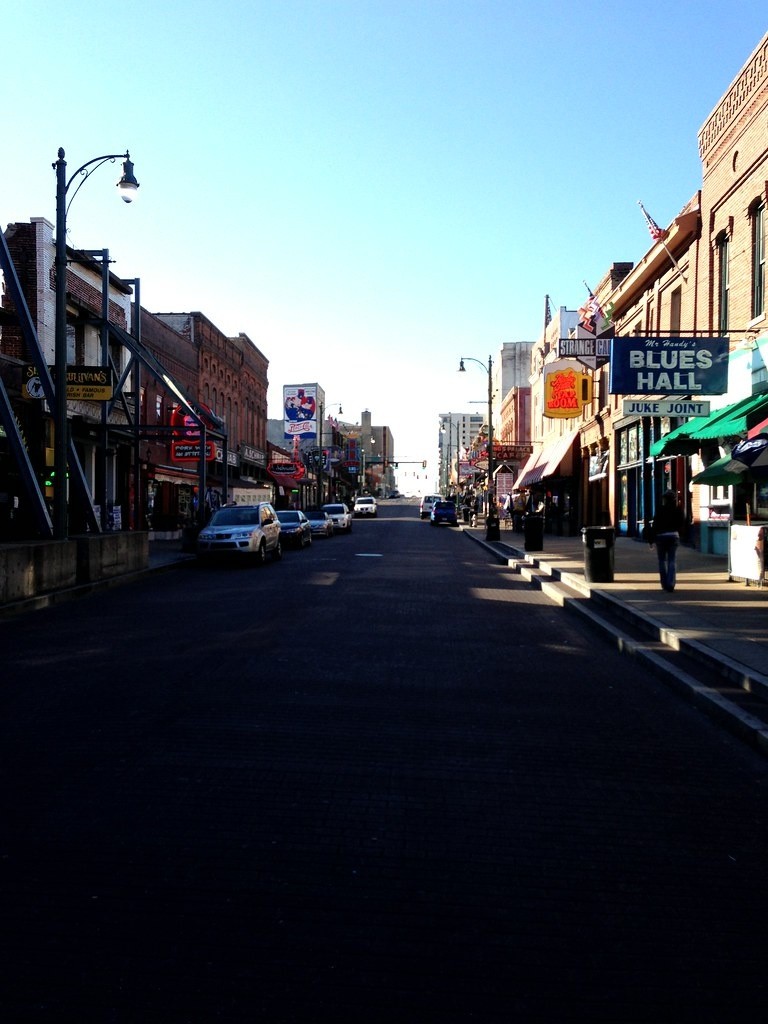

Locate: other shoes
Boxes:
[663,582,674,594]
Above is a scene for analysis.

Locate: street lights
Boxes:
[52,148,138,540]
[318,401,343,505]
[459,354,493,515]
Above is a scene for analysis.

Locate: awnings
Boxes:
[649,393,768,457]
[512,430,578,490]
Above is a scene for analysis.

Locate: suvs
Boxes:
[430,502,457,526]
[197,502,282,563]
[321,504,352,531]
[353,497,378,518]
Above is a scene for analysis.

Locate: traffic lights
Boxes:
[395,463,398,469]
[423,461,426,466]
[385,460,388,467]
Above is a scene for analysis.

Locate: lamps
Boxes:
[146,447,152,461]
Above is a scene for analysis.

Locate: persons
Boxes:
[653,491,684,593]
[513,490,526,513]
[503,492,510,520]
[473,497,479,515]
[465,492,472,507]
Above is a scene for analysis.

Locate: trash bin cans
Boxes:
[522,515,545,551]
[581,527,616,582]
[486,518,501,541]
[462,507,470,522]
[469,511,478,527]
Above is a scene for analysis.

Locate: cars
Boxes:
[274,510,312,547]
[303,512,334,538]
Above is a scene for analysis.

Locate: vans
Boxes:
[420,495,442,519]
